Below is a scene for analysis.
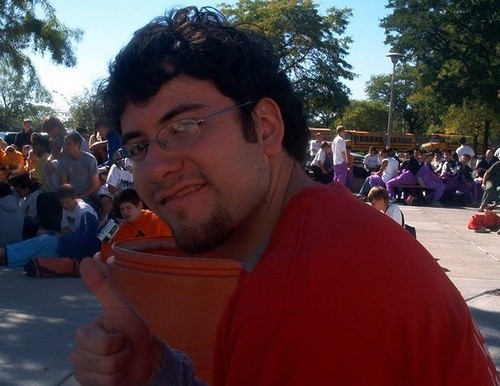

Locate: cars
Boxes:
[307,140,366,173]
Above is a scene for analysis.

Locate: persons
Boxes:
[0,116,500,278]
[70,5,500,386]
[331,126,348,187]
[367,186,405,230]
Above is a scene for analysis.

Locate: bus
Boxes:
[420,133,492,155]
[308,128,415,155]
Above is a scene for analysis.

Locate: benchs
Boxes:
[396,185,430,204]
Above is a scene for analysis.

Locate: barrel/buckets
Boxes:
[107,236,241,386]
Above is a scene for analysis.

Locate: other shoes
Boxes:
[431,202,443,207]
[389,198,397,203]
[487,203,495,210]
[478,205,484,212]
[425,194,432,202]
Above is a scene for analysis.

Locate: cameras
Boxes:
[96,217,119,242]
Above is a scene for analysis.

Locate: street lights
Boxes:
[385,52,406,148]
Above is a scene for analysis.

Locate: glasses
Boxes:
[111,100,255,171]
